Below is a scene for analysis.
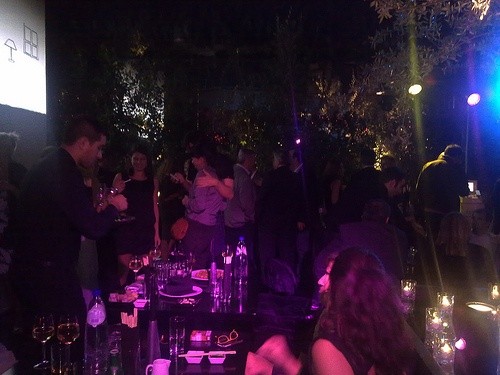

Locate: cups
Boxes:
[221,246,233,265]
[149,246,161,258]
[169,316,185,355]
[432,333,456,375]
[401,280,417,306]
[488,283,500,304]
[425,308,447,348]
[437,292,454,316]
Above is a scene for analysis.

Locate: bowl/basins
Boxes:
[208,351,225,363]
[185,351,204,363]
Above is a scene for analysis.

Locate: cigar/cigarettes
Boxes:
[124,179,132,183]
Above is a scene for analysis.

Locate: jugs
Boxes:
[146,359,171,375]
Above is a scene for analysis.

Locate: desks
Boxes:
[0,269,317,375]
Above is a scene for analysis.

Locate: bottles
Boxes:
[146,296,160,365]
[84,290,110,374]
[234,236,248,286]
[208,256,218,294]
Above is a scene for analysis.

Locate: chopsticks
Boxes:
[178,351,236,356]
[121,308,137,328]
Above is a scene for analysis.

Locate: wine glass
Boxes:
[129,256,143,281]
[54,315,80,373]
[31,313,55,369]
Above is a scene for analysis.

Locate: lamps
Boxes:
[465,301,495,320]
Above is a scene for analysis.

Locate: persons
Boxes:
[21,116,129,358]
[112,146,161,282]
[310,247,443,375]
[0,135,27,334]
[315,167,500,344]
[166,131,378,289]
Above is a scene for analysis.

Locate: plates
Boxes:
[191,269,224,280]
[159,286,202,297]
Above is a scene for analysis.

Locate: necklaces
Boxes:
[0,166,8,170]
